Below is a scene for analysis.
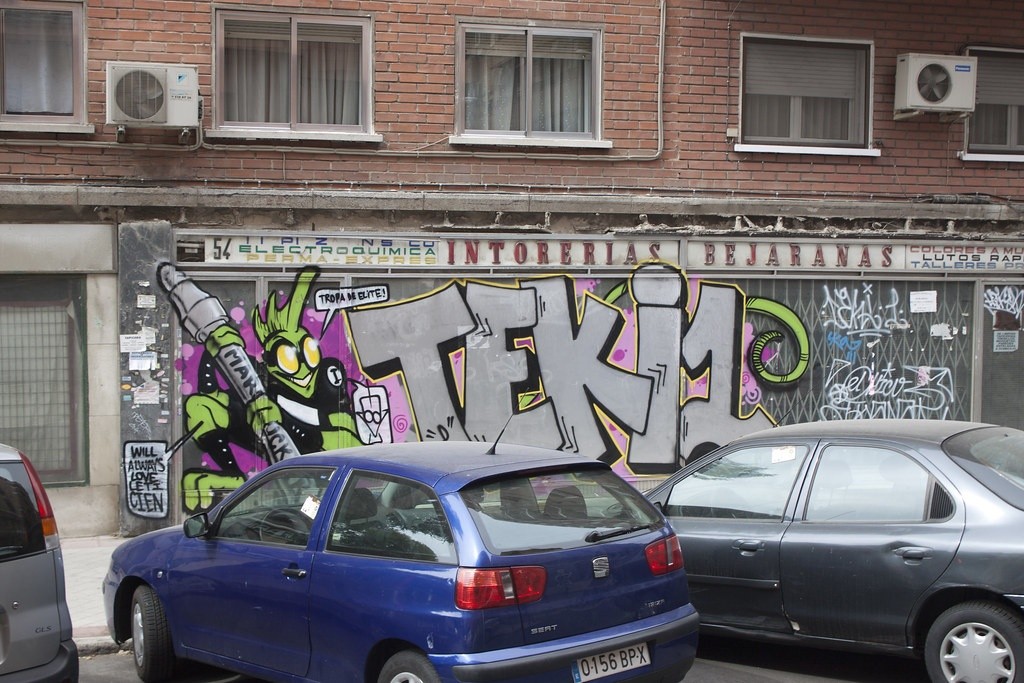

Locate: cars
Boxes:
[0,445,80,683]
[102,444,702,683]
[593,420,1024,683]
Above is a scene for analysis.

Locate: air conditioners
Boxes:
[105,60,200,133]
[895,52,979,115]
